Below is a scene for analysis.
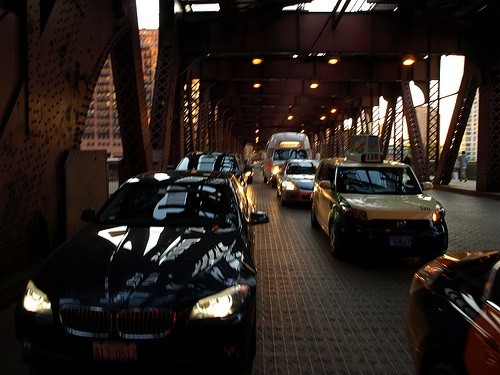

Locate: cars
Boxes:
[244,159,255,184]
[14,170,271,375]
[276,158,321,207]
[174,150,247,196]
[404,250,500,375]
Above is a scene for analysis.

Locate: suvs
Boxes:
[310,153,449,265]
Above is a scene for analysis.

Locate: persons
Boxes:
[403,154,411,174]
[458,150,468,182]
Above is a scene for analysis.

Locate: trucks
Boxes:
[263,132,313,189]
